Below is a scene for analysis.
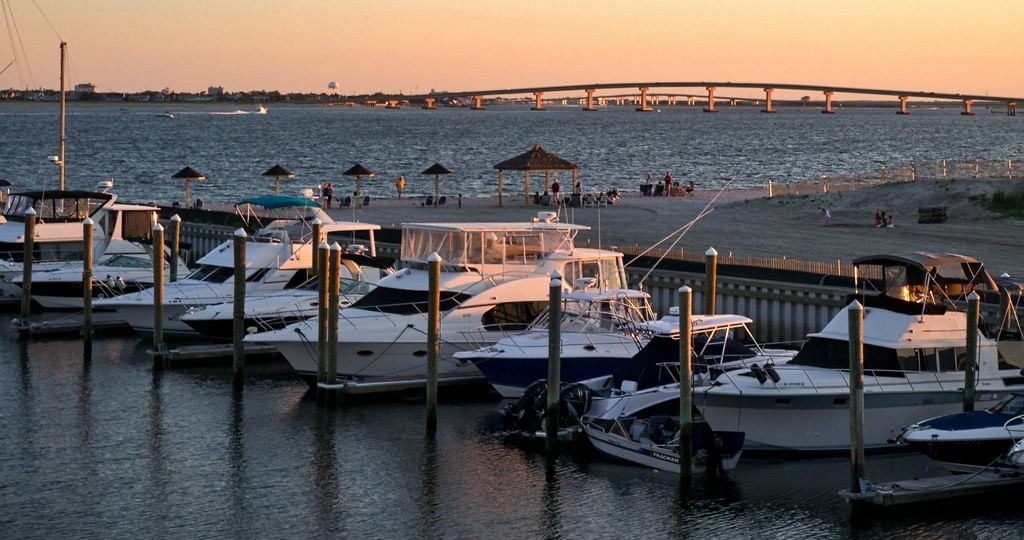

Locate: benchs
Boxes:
[916,207,947,223]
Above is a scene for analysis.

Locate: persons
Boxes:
[457,194,462,208]
[321,182,334,207]
[575,181,584,196]
[338,237,349,254]
[644,173,694,197]
[551,178,561,204]
[395,175,406,198]
[822,207,830,224]
[337,190,358,207]
[664,171,672,197]
[875,209,893,227]
[192,199,203,208]
[598,187,619,204]
[534,190,550,207]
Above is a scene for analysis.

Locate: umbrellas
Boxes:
[171,165,206,206]
[262,164,295,195]
[422,162,453,204]
[342,164,374,195]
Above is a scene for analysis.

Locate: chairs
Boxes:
[172,201,181,208]
[595,196,608,207]
[687,187,693,195]
[422,196,433,207]
[362,195,369,206]
[653,185,663,196]
[530,195,540,207]
[341,201,352,208]
[639,185,652,195]
[584,196,594,209]
[571,194,580,206]
[434,196,446,206]
[540,196,551,208]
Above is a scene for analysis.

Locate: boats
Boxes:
[580,414,746,492]
[654,252,1024,460]
[887,390,1024,475]
[0,42,807,440]
